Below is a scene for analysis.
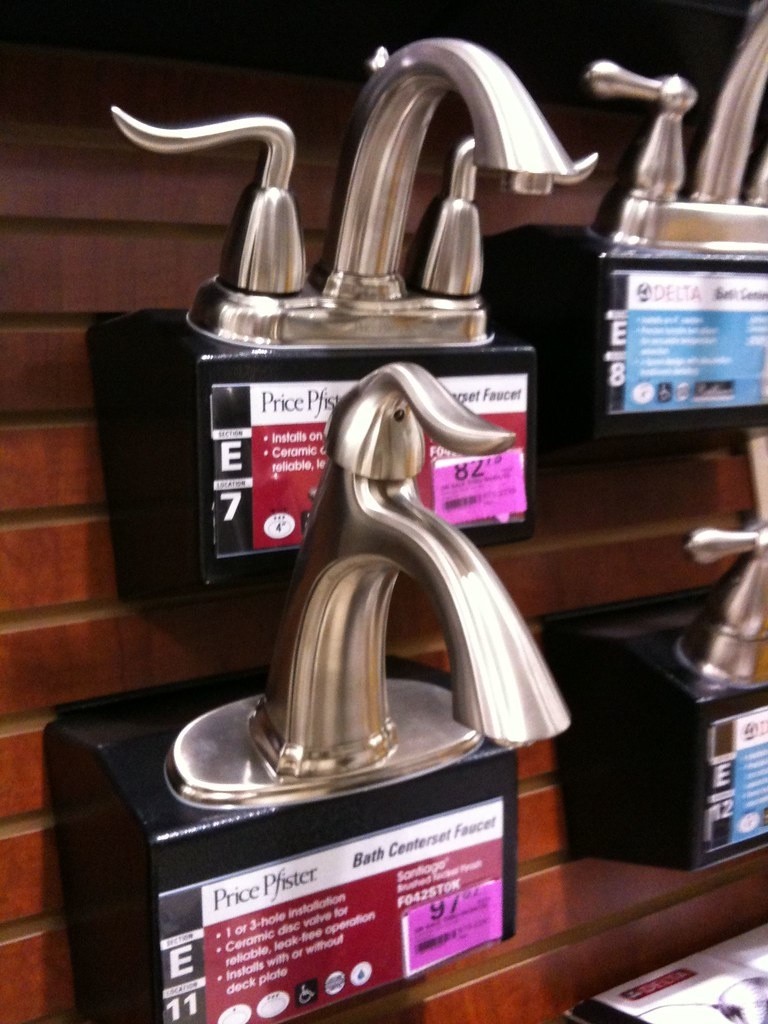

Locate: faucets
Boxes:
[311,36,577,349]
[161,360,574,813]
[689,0,768,206]
